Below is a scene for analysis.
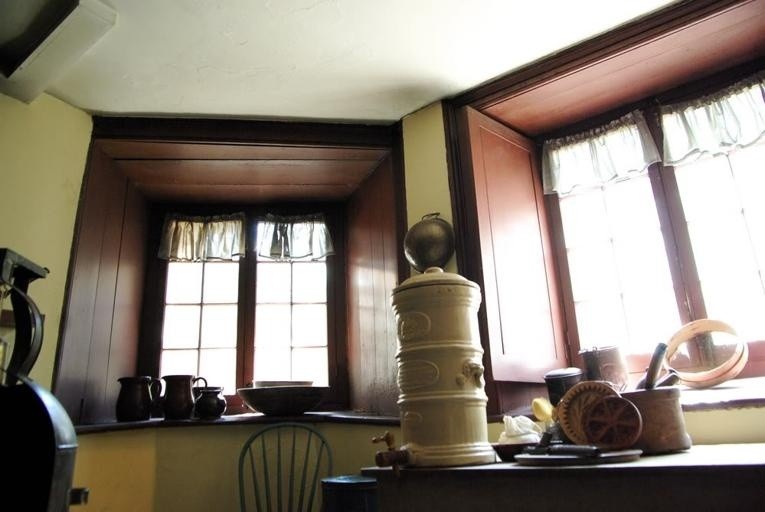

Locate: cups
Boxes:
[164,374,208,420]
[114,375,164,424]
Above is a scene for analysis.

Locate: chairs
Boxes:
[236,423,332,512]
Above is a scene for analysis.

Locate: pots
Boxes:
[402,212,453,270]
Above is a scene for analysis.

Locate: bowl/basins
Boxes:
[237,380,331,413]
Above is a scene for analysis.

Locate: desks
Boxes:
[359,441,765,512]
